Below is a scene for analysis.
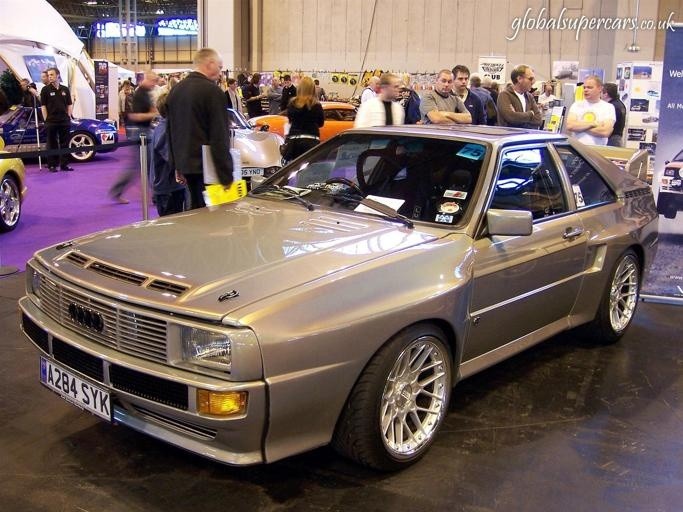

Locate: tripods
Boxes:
[14,96,46,171]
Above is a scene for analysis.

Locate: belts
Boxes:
[290,134,319,140]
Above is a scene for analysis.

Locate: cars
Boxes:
[17,123,661,480]
[1,136,29,232]
[656,148,683,218]
[1,101,119,159]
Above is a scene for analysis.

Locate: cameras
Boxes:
[27,83,36,90]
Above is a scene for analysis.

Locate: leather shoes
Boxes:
[48,166,58,172]
[61,165,73,171]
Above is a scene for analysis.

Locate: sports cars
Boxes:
[149,104,290,194]
[246,99,367,162]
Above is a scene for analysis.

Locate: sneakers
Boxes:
[117,196,129,204]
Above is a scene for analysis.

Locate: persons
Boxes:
[19,48,626,219]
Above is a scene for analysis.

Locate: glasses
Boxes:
[521,75,535,81]
